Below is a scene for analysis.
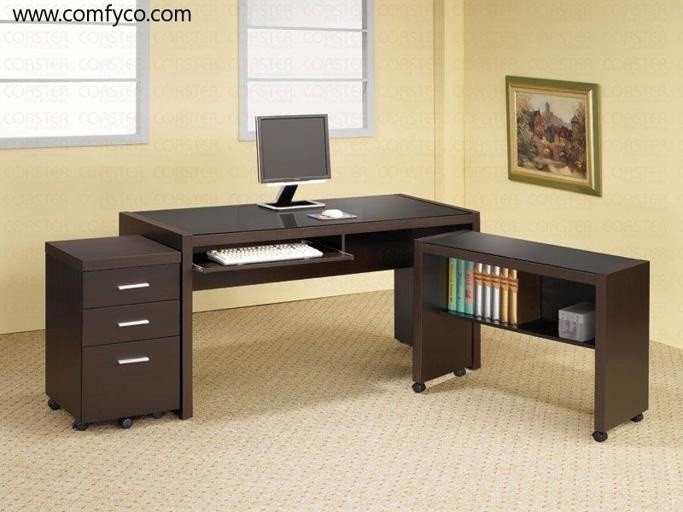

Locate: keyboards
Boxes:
[206,241,323,266]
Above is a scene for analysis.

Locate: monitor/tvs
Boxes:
[255,113,331,212]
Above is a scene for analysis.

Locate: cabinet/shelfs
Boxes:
[43,233,181,424]
[411,228,649,433]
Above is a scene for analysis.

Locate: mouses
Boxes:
[322,209,344,218]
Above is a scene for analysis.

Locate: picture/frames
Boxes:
[504,74,603,199]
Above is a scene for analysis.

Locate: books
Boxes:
[508,269,541,326]
[473,263,482,317]
[464,261,474,314]
[447,257,456,311]
[490,266,500,321]
[482,264,491,319]
[455,259,464,313]
[499,268,508,323]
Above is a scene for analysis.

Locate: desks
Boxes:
[117,193,482,421]
